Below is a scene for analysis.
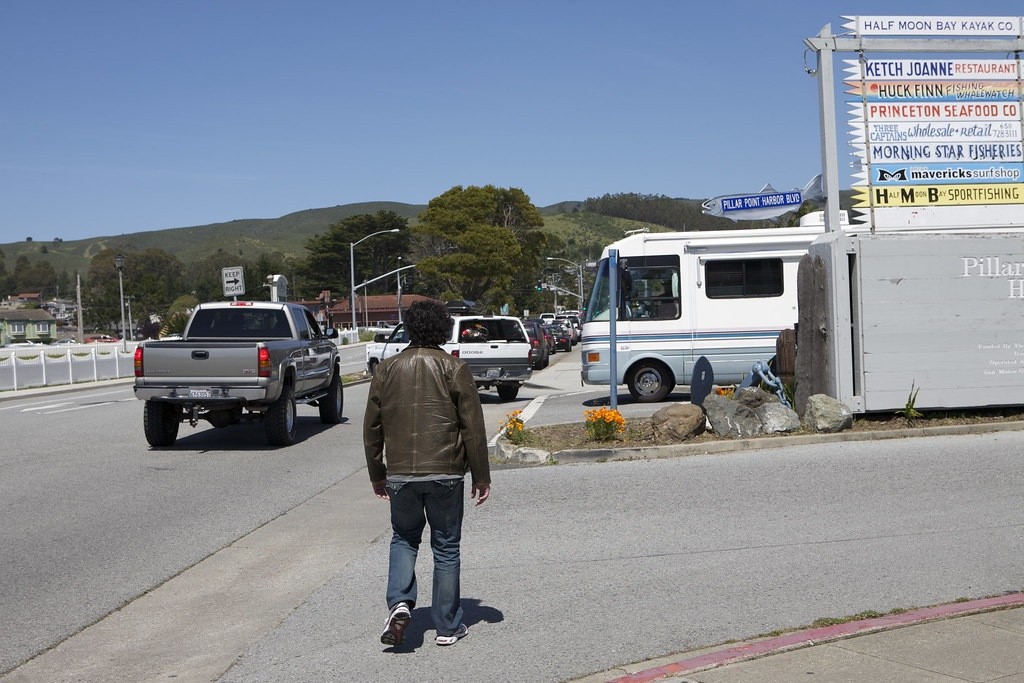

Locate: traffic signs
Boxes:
[720,191,802,212]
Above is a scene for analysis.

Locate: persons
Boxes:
[363,299,492,645]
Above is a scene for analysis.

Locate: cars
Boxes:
[522,306,582,370]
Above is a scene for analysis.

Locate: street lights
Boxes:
[114,253,127,353]
[547,257,584,311]
[350,228,400,330]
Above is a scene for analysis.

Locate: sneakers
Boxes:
[435,623,468,644]
[380,601,411,645]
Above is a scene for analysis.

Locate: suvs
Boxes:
[365,306,535,402]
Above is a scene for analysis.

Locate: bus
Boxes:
[580,210,866,403]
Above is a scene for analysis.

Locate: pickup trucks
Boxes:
[133,300,343,447]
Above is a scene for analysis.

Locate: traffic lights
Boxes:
[536,278,543,292]
